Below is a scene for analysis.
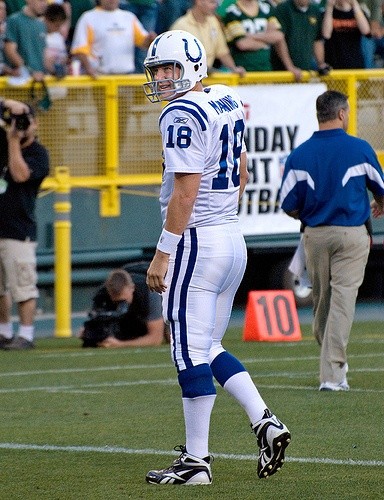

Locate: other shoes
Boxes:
[0,334,14,348]
[319,378,350,392]
[3,337,36,351]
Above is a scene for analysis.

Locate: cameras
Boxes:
[13,113,30,130]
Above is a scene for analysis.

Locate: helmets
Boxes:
[141,29,208,103]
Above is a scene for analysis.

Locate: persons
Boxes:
[79,262,170,348]
[142,30,291,485]
[0,1,384,177]
[0,96,50,350]
[279,90,384,391]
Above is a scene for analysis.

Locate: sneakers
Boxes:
[146,444,215,486]
[249,409,292,479]
